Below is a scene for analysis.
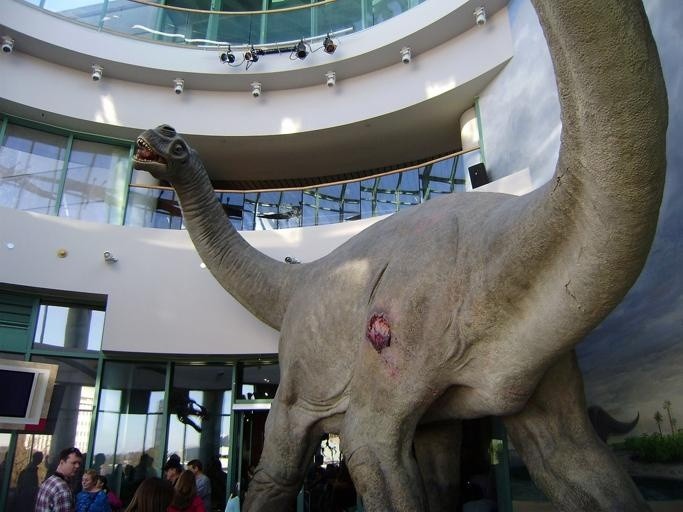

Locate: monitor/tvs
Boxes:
[0,365,39,418]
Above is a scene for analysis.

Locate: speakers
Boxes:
[468,163,489,189]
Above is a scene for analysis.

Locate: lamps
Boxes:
[89,63,103,81]
[472,6,488,26]
[248,81,262,97]
[172,77,184,95]
[103,251,119,264]
[0,35,15,55]
[324,70,335,88]
[399,43,412,65]
[216,33,340,67]
[285,256,301,265]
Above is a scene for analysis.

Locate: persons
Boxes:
[17,452,43,512]
